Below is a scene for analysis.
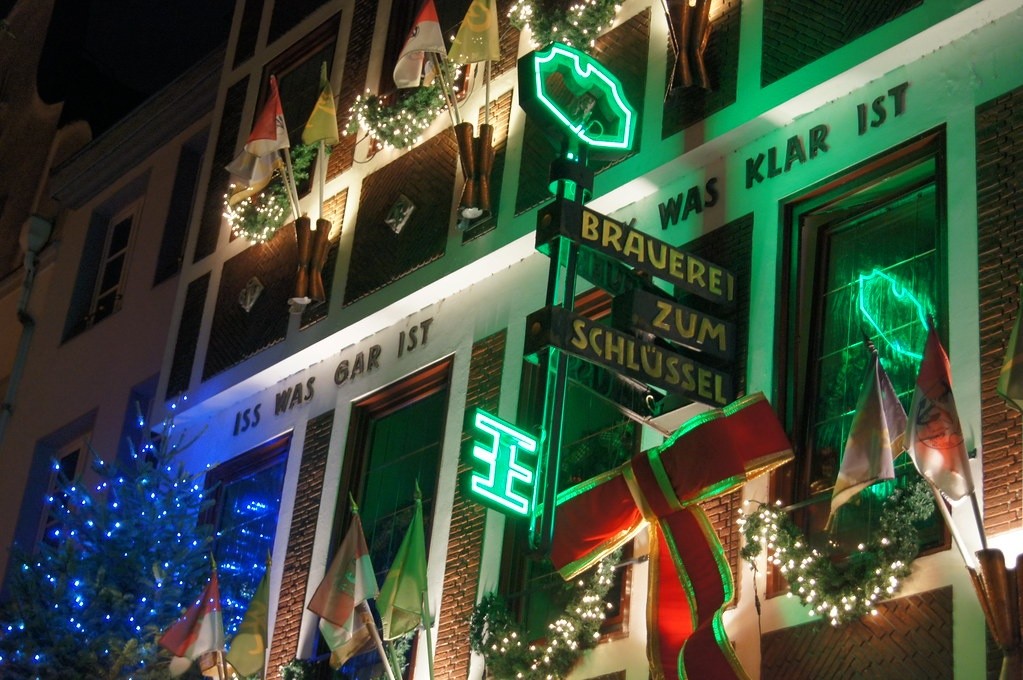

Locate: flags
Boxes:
[902,326,975,502]
[226,566,268,676]
[300,63,338,146]
[306,516,379,631]
[392,0,446,89]
[447,0,500,64]
[319,599,378,670]
[375,501,427,640]
[423,51,439,87]
[160,572,226,661]
[997,284,1022,415]
[224,148,281,185]
[242,77,290,158]
[822,354,907,529]
